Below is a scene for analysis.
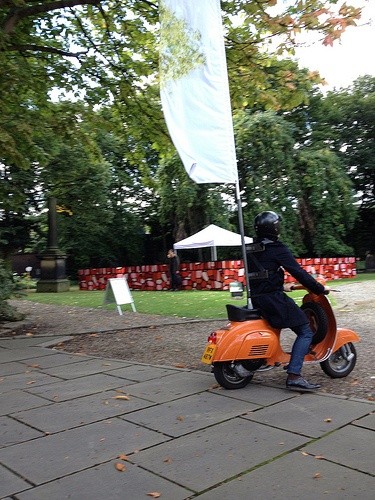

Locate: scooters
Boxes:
[200,276,361,391]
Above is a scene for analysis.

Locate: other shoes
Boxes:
[175,289,178,291]
[170,288,173,291]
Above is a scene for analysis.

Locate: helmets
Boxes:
[254,211,283,238]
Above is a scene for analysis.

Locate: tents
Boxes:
[173,224,254,262]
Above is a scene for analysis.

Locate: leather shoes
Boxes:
[286,376,322,391]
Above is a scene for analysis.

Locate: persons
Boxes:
[167,250,181,292]
[242,211,326,392]
